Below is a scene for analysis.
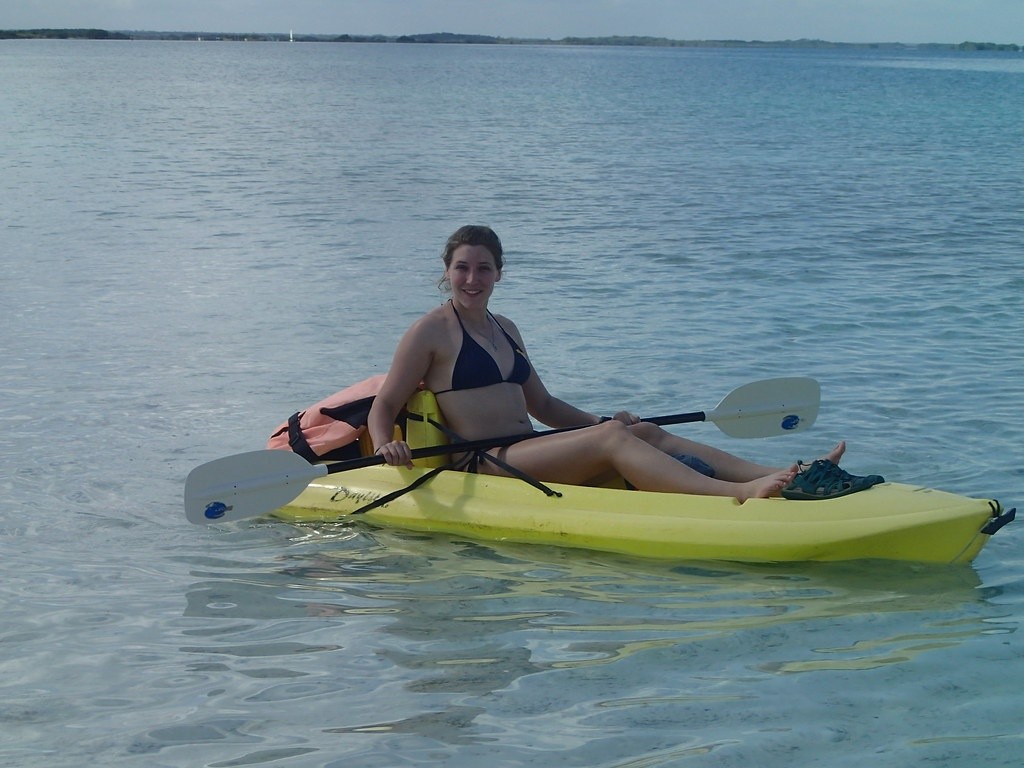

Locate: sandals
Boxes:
[781,460,873,500]
[809,459,885,485]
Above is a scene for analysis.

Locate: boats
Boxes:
[261,391,1019,568]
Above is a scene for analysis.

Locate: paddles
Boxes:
[182,376,823,528]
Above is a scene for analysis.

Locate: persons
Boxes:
[367,225,846,503]
